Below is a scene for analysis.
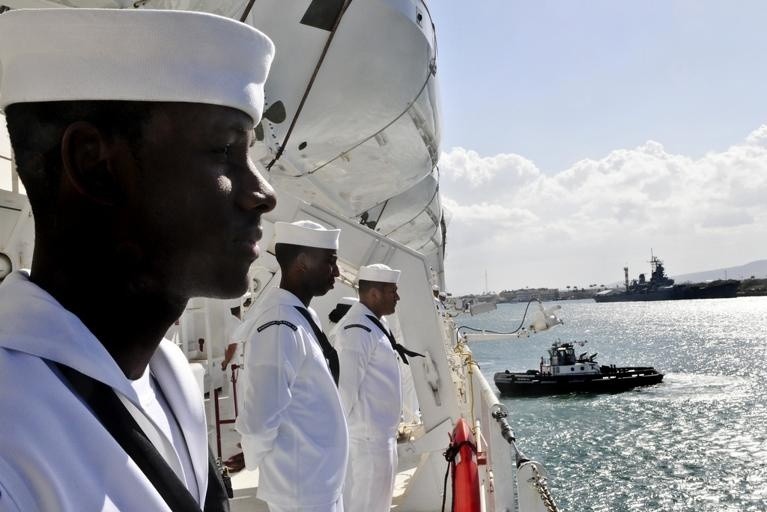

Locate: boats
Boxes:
[591,245,742,304]
[493,338,667,398]
[1,0,562,512]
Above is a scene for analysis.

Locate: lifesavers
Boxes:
[450,420,481,511]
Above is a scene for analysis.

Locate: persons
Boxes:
[221,301,245,418]
[234,220,357,511]
[330,264,405,511]
[431,284,448,317]
[328,297,358,323]
[1,8,274,511]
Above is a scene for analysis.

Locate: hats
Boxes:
[1,6,276,129]
[274,219,341,252]
[439,292,446,297]
[359,263,401,284]
[432,285,440,290]
